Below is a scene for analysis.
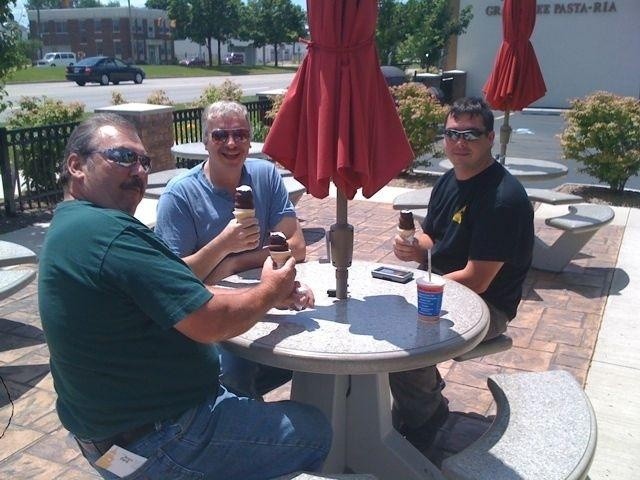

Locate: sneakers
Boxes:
[400,394,449,452]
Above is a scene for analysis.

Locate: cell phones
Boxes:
[372,266,414,283]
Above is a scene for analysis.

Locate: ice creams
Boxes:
[266,231,291,269]
[398,211,416,242]
[231,185,255,222]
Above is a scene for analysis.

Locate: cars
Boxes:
[65,56,147,86]
[178,56,206,67]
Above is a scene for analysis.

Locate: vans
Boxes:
[37,52,75,69]
[222,52,246,65]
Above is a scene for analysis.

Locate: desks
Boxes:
[168,139,269,164]
[213,257,495,479]
[437,154,571,183]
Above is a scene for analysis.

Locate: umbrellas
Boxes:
[482,0,547,166]
[261,0,414,301]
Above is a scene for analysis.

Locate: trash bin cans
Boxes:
[412,70,467,107]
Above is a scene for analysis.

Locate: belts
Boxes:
[73,419,167,453]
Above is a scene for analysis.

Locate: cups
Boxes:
[416,275,446,325]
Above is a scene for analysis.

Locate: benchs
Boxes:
[393,180,588,223]
[132,164,313,232]
[545,200,615,276]
[0,236,40,309]
[442,368,599,479]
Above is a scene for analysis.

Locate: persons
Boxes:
[37,113,333,480]
[153,100,306,399]
[389,95,535,453]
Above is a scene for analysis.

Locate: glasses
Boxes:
[82,146,150,171]
[210,127,250,145]
[445,127,482,142]
